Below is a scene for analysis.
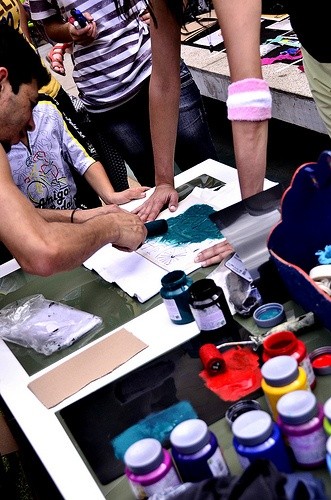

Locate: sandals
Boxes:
[46,42,71,74]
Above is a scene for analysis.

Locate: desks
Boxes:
[0,159,277,500]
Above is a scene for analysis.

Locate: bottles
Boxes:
[232,410,298,472]
[159,270,195,324]
[122,438,181,500]
[262,332,315,389]
[274,390,331,476]
[260,356,314,415]
[170,419,230,484]
[186,278,234,340]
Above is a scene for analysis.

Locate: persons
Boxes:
[0,0,330,278]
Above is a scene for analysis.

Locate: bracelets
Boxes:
[70,208,77,223]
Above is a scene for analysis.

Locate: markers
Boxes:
[68,9,86,29]
